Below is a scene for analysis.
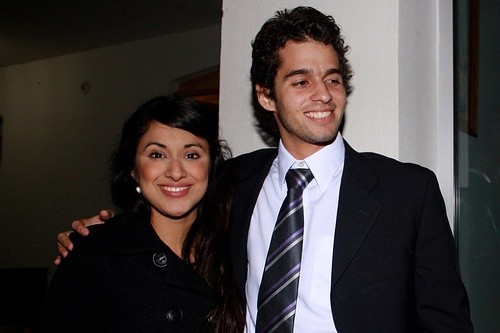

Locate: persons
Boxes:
[53,95,248,333]
[53,5,474,333]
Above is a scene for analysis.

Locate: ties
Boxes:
[255,168,314,333]
